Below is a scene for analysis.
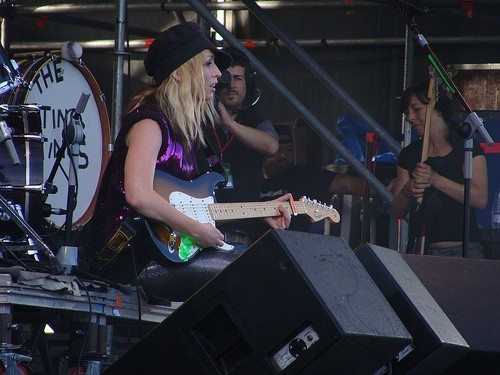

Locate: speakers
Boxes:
[103,227,500,374]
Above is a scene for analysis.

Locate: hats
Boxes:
[273,124,293,144]
[215,47,258,102]
[144,21,232,87]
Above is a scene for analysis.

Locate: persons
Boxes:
[259,125,366,235]
[392,80,488,258]
[91,21,291,301]
[194,51,280,239]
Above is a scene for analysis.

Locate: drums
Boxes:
[0,53,112,232]
[0,43,20,95]
[0,102,45,244]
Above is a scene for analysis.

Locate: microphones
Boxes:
[43,204,66,217]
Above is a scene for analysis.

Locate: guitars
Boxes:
[142,169,341,263]
[405,64,442,256]
